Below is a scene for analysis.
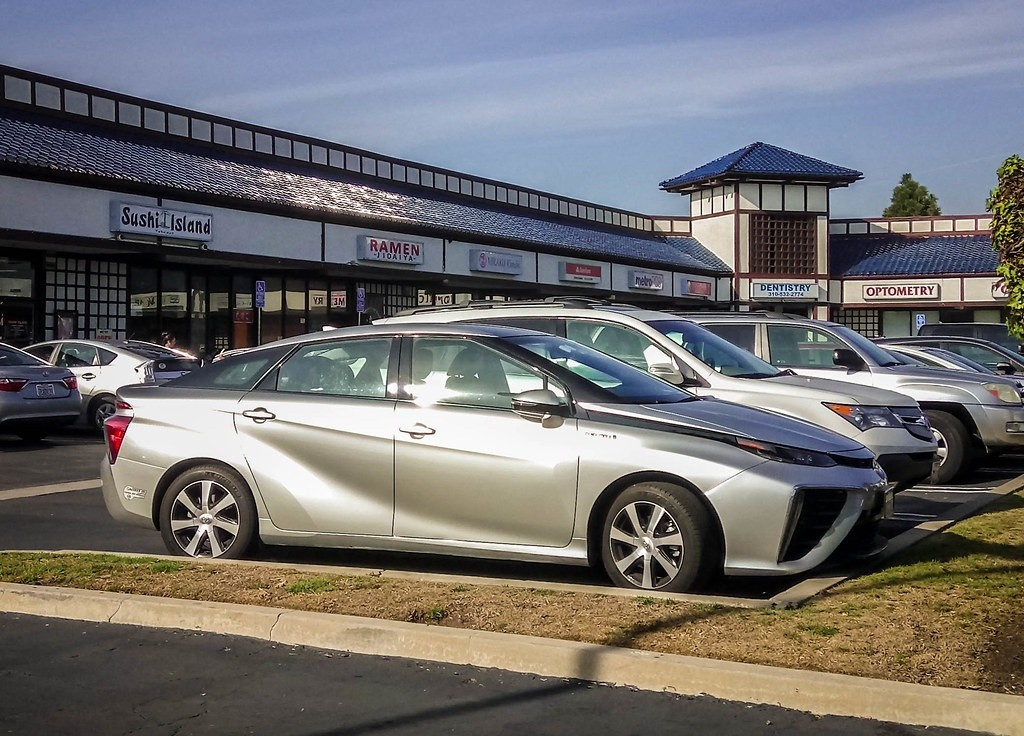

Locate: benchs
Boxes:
[283,354,386,396]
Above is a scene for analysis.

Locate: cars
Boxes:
[100,322,887,594]
[19,338,202,436]
[0,342,83,441]
[869,335,1023,379]
[875,342,1023,401]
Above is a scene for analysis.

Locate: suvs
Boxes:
[656,311,1024,485]
[372,296,937,495]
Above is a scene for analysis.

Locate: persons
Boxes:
[162,332,177,349]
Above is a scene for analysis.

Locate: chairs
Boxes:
[413,347,443,401]
[444,347,497,398]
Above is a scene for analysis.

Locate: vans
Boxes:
[914,323,1024,358]
